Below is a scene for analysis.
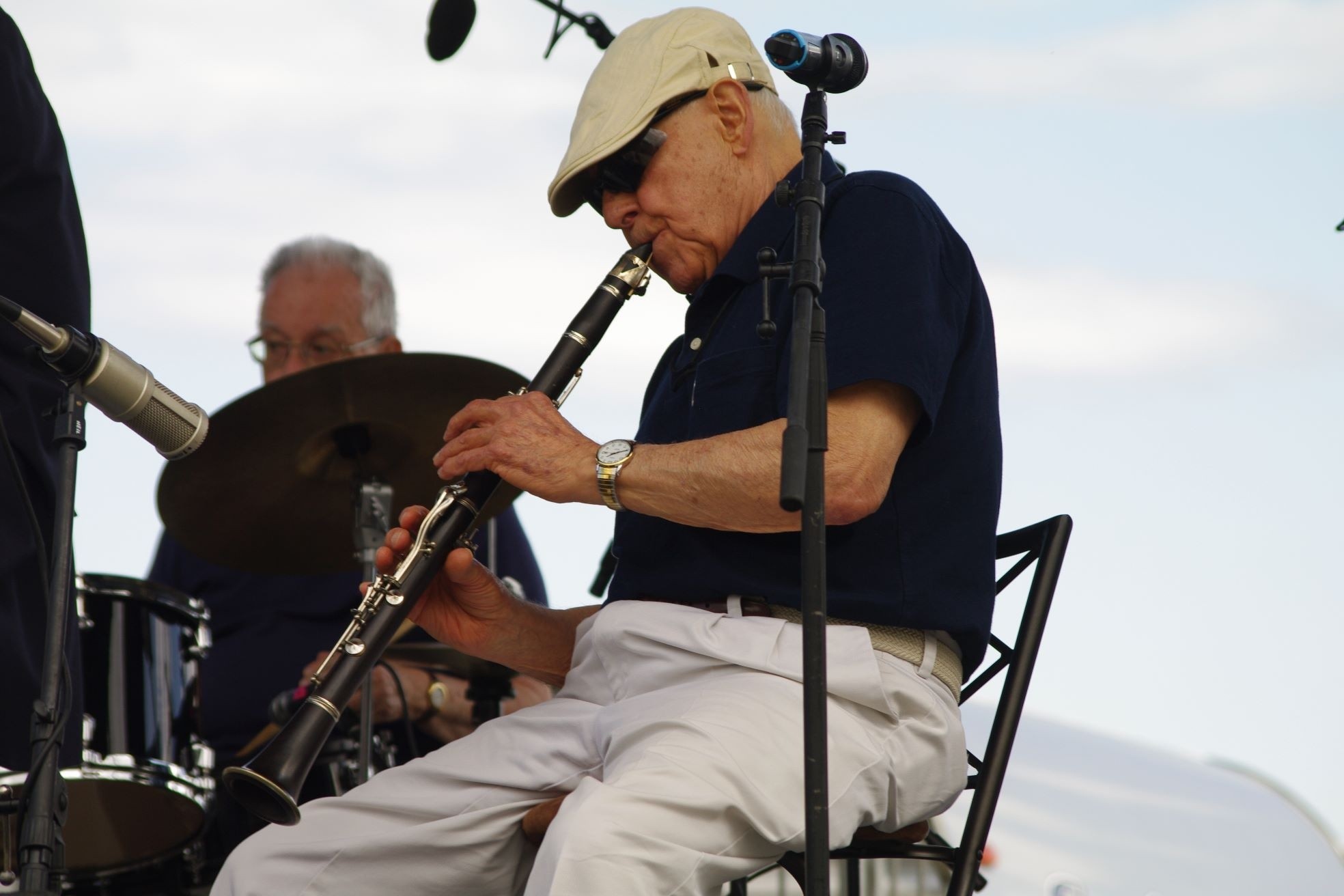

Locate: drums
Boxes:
[0,570,210,887]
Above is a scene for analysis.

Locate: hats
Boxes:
[547,6,777,218]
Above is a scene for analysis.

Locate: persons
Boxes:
[152,235,548,802]
[212,7,1003,896]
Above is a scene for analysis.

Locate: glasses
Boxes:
[247,332,382,365]
[575,82,765,217]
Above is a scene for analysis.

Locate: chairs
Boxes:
[722,514,1072,896]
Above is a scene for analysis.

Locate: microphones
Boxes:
[764,28,869,95]
[1,296,211,466]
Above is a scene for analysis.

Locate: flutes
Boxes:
[218,234,651,827]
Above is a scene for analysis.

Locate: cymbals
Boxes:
[152,353,529,567]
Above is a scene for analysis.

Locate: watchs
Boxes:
[411,667,449,728]
[595,440,640,513]
